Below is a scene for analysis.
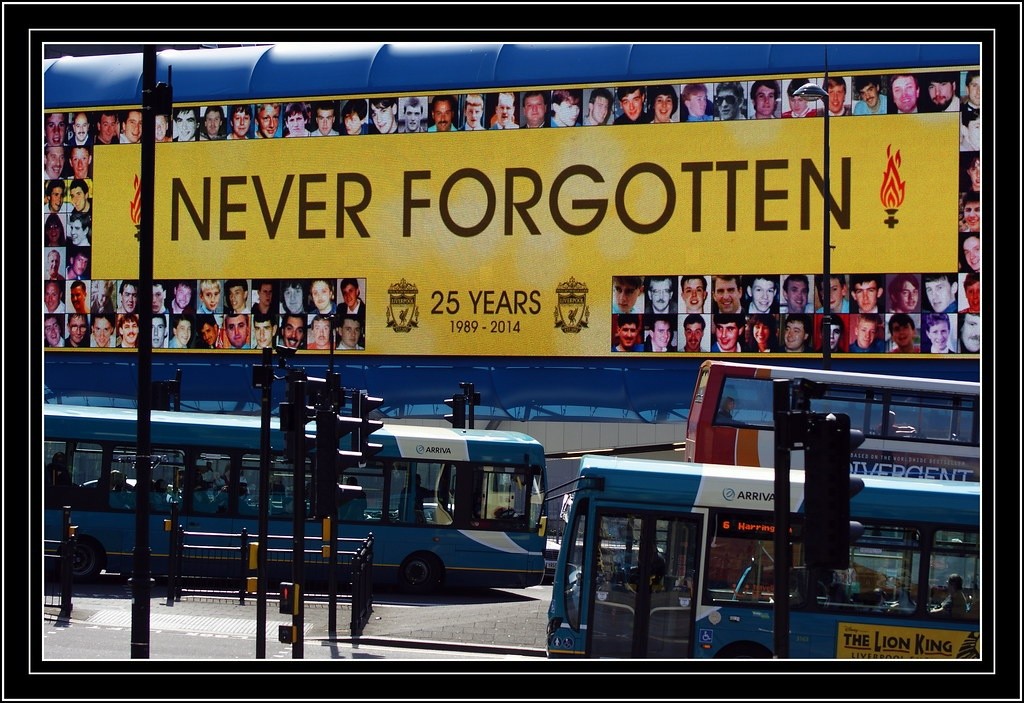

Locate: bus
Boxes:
[44,403,549,594]
[546,454,982,659]
[685,359,980,482]
[574,541,665,572]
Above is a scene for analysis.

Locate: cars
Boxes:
[544,540,561,582]
[728,559,888,606]
[881,578,926,599]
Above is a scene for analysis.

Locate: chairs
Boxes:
[53,474,294,514]
[706,569,980,619]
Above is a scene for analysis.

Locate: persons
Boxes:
[340,477,367,519]
[46,452,72,486]
[396,474,435,519]
[114,470,133,491]
[45,70,980,355]
[220,464,230,484]
[878,411,915,437]
[607,537,665,586]
[930,574,967,614]
[194,471,208,491]
[719,397,735,418]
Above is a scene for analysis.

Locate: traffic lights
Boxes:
[314,410,362,520]
[443,393,466,429]
[278,581,299,616]
[353,389,384,463]
